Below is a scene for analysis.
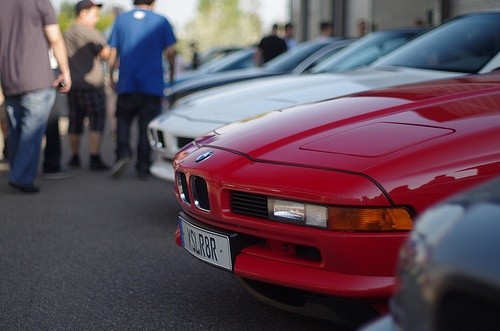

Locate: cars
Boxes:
[173,71,500,297]
[165,24,429,104]
[148,9,500,185]
[391,177,499,330]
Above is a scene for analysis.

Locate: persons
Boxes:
[318,20,335,37]
[0,0,74,196]
[255,23,288,67]
[281,23,299,50]
[184,40,202,71]
[105,0,178,179]
[357,21,368,37]
[61,0,114,171]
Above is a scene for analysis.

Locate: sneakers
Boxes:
[8,180,40,193]
[43,165,76,179]
[108,154,131,178]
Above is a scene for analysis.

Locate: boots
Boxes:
[67,152,81,167]
[89,153,112,172]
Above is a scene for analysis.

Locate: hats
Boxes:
[77,1,103,13]
